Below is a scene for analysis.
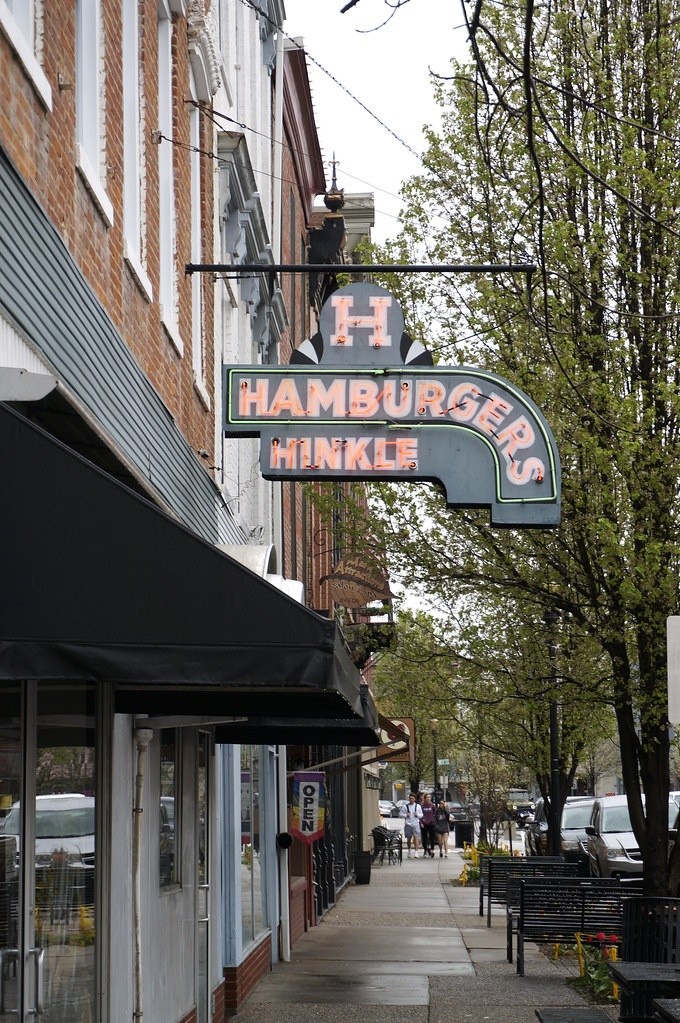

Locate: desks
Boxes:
[604,962,679,1023]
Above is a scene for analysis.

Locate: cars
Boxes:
[379,800,410,818]
[445,802,480,820]
[524,791,680,878]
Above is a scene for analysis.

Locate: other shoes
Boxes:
[439,850,448,858]
[430,852,435,858]
[406,850,419,858]
[424,851,428,858]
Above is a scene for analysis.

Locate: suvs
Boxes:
[484,789,535,828]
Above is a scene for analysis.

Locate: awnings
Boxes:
[0,400,381,745]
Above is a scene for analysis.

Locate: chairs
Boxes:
[368,826,402,866]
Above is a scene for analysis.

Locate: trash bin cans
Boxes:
[453,820,475,849]
[619,896,680,1023]
[353,851,371,885]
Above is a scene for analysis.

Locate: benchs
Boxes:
[476,854,644,977]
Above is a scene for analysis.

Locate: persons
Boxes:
[398,792,450,858]
